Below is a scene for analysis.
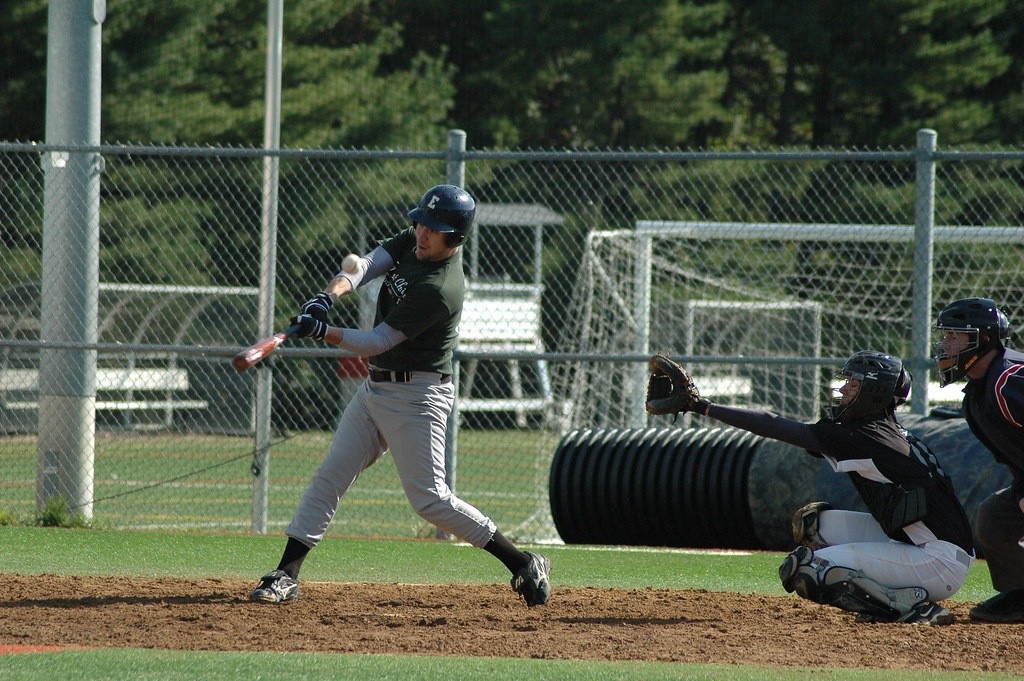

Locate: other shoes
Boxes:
[969,590,1024,622]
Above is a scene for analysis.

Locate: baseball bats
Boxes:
[231,321,306,374]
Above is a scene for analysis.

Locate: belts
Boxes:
[368,369,450,383]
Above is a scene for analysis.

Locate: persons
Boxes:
[645,351,976,624]
[248,184,551,606]
[931,297,1024,622]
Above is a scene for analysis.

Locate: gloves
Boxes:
[300,292,333,322]
[288,313,327,341]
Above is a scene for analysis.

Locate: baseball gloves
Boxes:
[646,354,705,420]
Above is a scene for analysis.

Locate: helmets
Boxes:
[826,349,912,421]
[407,184,476,247]
[933,298,1010,389]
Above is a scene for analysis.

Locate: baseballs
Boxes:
[341,253,364,277]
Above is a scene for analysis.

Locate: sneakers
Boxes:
[251,569,299,606]
[511,550,551,608]
[905,602,955,627]
